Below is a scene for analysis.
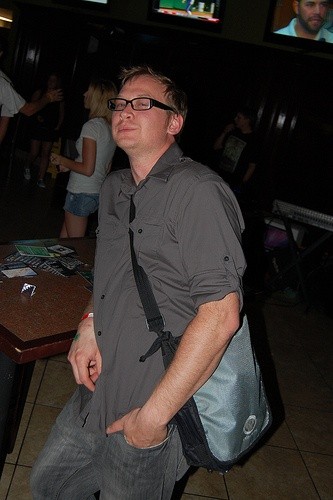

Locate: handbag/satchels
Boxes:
[157,315,272,469]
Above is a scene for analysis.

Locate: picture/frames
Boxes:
[50,0,113,13]
[263,0,333,54]
[147,0,227,33]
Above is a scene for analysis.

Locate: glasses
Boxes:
[107,97,178,116]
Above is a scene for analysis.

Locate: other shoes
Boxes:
[37,177,45,187]
[23,168,31,179]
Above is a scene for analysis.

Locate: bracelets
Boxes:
[80,312,94,320]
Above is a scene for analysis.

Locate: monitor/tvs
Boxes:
[148,0,226,30]
[264,0,333,50]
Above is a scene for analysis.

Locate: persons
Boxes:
[29,64,250,500]
[272,0,333,44]
[24,73,64,188]
[50,79,118,239]
[0,33,63,142]
[182,0,195,16]
[212,109,258,198]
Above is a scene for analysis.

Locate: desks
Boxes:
[0,237,97,365]
[265,194,333,312]
